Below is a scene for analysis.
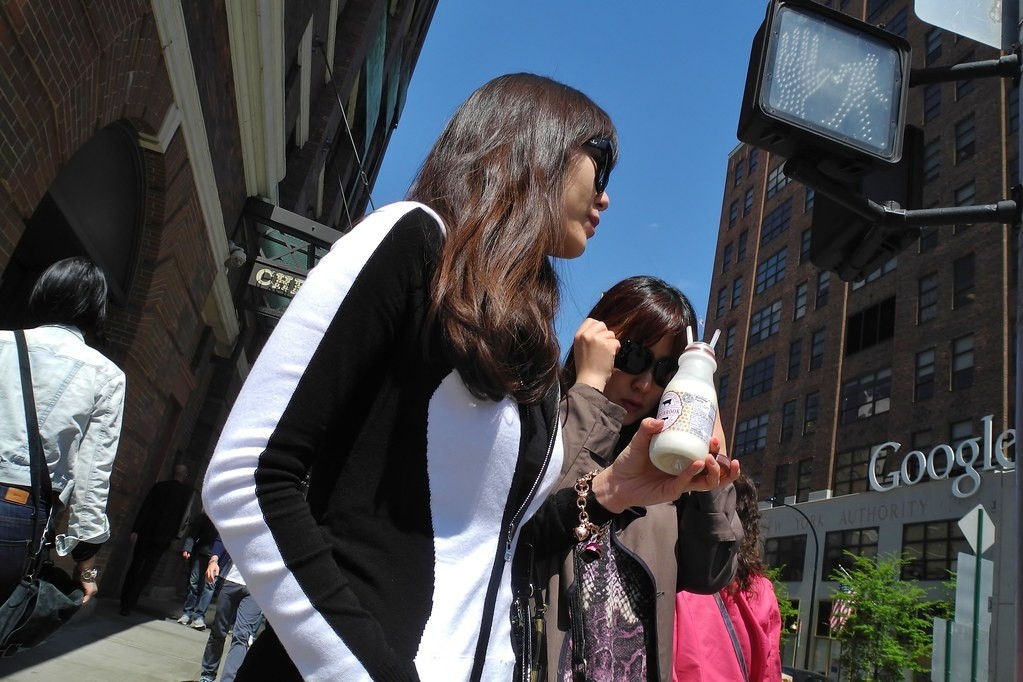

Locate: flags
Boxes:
[829,570,857,638]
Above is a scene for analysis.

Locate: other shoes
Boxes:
[120,608,129,617]
[200,676,214,682]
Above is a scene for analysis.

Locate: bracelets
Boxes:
[73,565,98,580]
[208,560,218,564]
[573,468,612,563]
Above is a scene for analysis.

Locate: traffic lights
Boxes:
[773,124,926,282]
[737,0,915,167]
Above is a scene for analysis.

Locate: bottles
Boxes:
[649,342,718,477]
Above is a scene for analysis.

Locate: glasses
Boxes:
[614,339,679,389]
[587,135,613,196]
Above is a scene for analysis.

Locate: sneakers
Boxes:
[190,618,206,631]
[178,615,191,625]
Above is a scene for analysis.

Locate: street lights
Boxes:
[766,497,819,670]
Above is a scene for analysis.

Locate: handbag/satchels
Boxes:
[530,615,545,682]
[0,566,85,662]
[562,470,649,682]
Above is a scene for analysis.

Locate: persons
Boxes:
[672,471,783,682]
[200,533,263,681]
[512,276,749,681]
[118,463,188,617]
[200,72,741,682]
[0,256,127,658]
[177,512,220,630]
